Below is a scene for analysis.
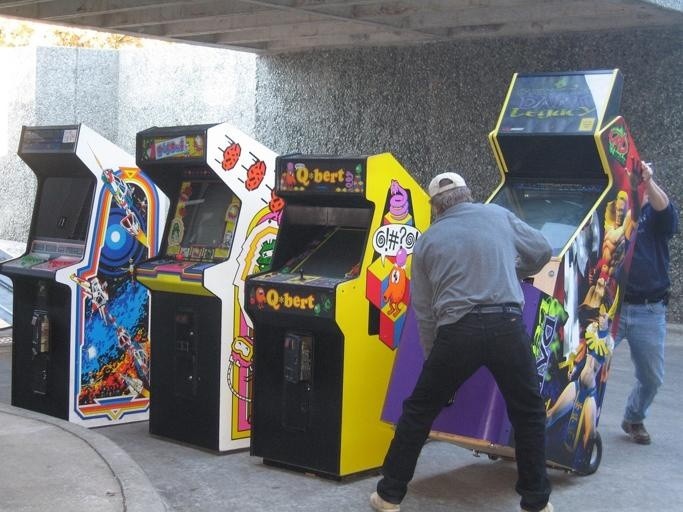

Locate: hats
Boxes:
[429,172,466,198]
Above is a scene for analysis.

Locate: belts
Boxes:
[623,293,663,305]
[466,306,522,315]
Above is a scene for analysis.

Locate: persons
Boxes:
[370,172,553,512]
[545,156,643,470]
[614,161,679,445]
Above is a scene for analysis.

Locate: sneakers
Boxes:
[519,501,553,512]
[621,419,650,445]
[370,491,400,512]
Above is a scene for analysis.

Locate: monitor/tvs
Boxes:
[274,223,368,279]
[164,179,241,260]
[490,182,605,257]
[34,176,95,245]
